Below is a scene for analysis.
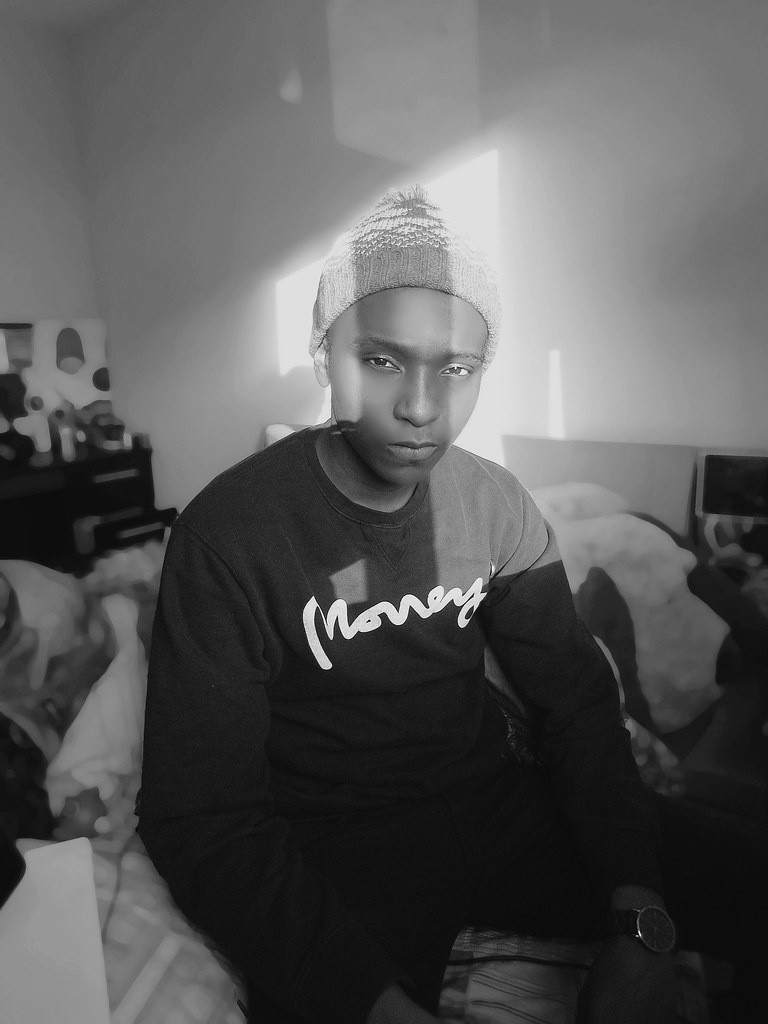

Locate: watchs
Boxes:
[604,906,677,952]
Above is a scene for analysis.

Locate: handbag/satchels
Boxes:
[436,925,735,1024]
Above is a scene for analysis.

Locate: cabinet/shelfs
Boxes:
[1,432,178,574]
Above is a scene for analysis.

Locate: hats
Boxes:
[309,194,502,377]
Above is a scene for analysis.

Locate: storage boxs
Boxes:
[695,449,768,550]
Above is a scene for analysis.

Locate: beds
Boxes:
[0,424,702,1024]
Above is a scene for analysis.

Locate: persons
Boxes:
[134,181,680,1024]
